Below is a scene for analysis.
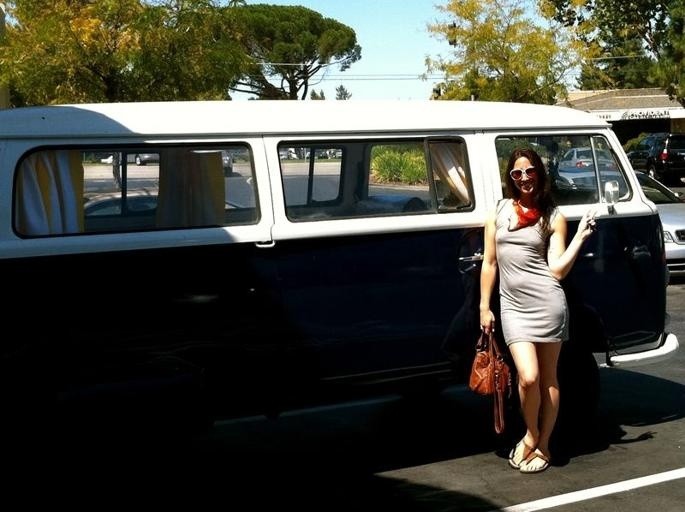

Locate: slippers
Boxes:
[508,438,549,472]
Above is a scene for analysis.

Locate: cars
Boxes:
[107,153,159,166]
[550,164,684,286]
[557,147,617,173]
[278,148,342,163]
[222,149,234,175]
[83,186,243,219]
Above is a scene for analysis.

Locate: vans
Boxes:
[0,98,679,458]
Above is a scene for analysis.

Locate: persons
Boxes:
[478,147,596,472]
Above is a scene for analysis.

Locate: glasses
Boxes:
[510,167,537,180]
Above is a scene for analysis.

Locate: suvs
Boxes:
[627,131,684,185]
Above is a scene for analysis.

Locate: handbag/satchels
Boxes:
[470,330,511,396]
